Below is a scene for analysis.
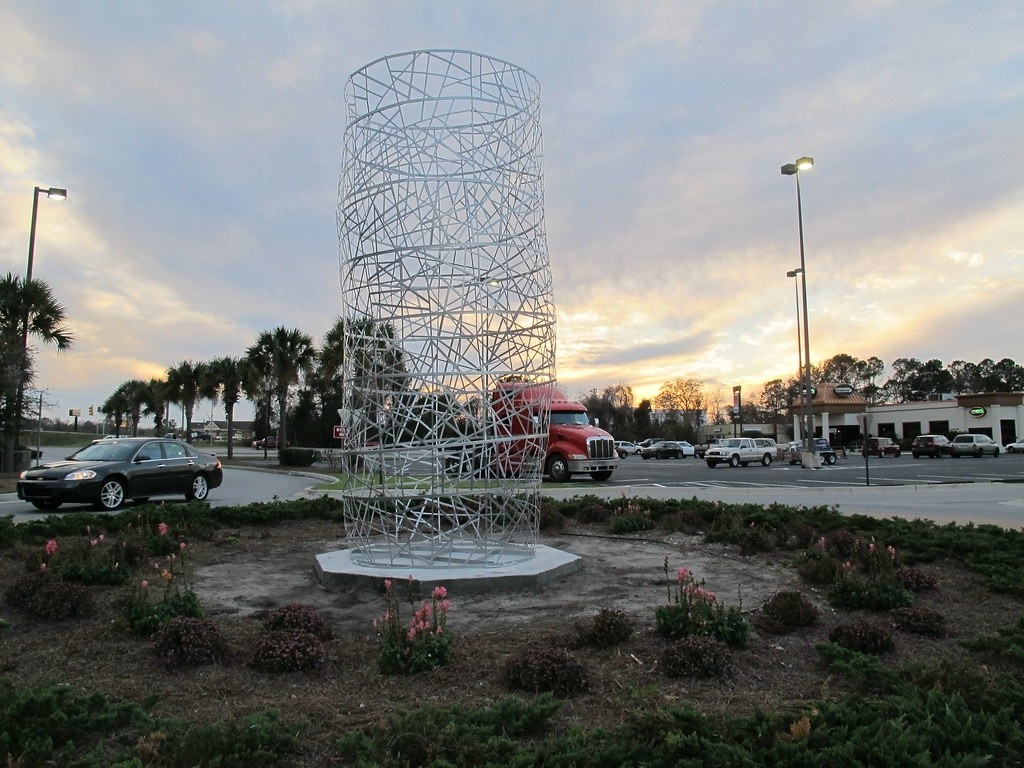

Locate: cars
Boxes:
[613,441,644,454]
[950,434,1000,458]
[636,438,664,448]
[862,437,900,458]
[911,434,950,458]
[668,441,699,458]
[640,442,684,460]
[90,434,129,444]
[1005,438,1024,453]
[694,437,720,459]
[15,437,223,512]
[789,438,836,465]
[252,436,290,450]
[613,446,629,459]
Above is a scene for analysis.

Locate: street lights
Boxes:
[785,269,808,452]
[780,156,819,456]
[13,188,65,463]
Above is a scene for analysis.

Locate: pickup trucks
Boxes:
[704,438,777,467]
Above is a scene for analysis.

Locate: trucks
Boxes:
[437,377,619,484]
